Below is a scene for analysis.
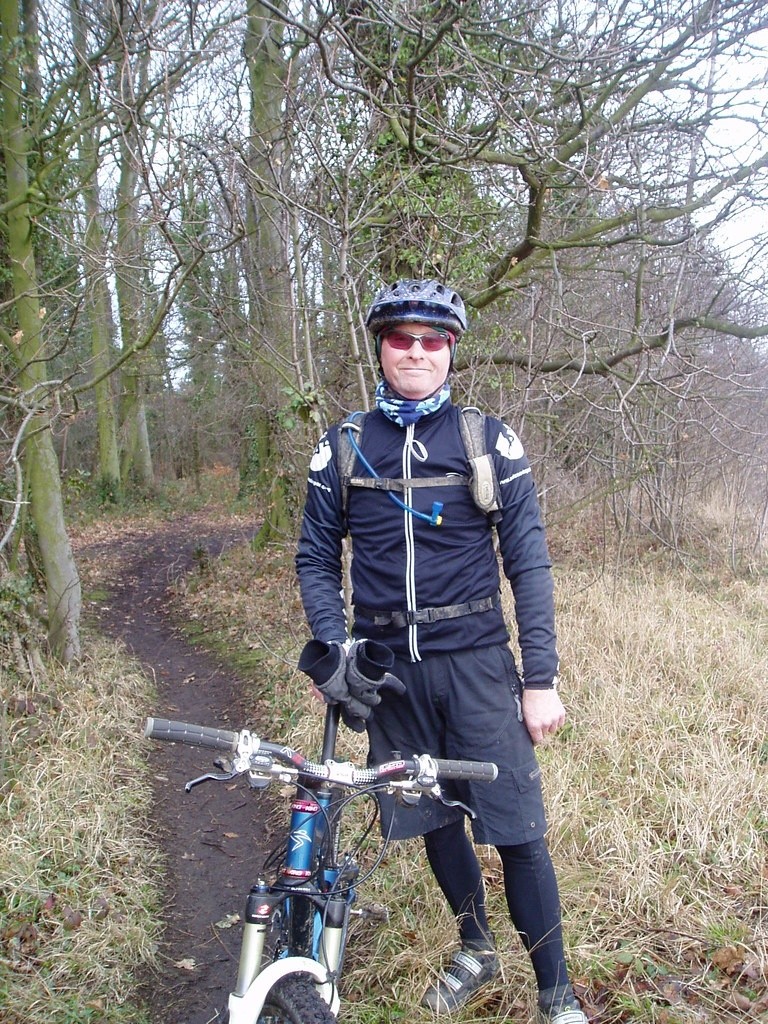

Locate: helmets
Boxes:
[365,277,467,344]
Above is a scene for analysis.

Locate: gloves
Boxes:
[298,638,405,734]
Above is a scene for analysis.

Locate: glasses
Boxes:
[381,330,450,351]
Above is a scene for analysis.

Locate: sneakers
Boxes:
[538,985,588,1024]
[421,947,497,1014]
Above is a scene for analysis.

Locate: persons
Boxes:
[295,282,591,1024]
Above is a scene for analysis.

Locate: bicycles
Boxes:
[142,702,500,1024]
